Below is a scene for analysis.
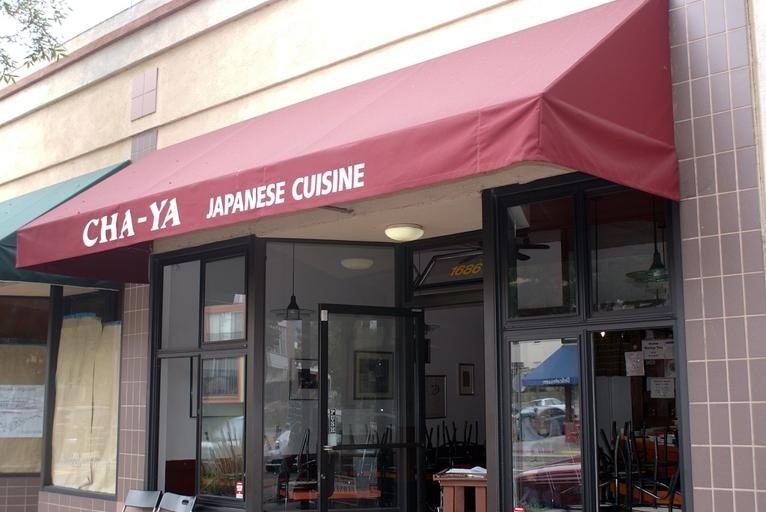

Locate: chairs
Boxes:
[121,487,197,512]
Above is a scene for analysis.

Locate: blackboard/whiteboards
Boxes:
[1,322,123,502]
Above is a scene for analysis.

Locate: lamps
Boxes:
[285,243,302,320]
[383,223,425,243]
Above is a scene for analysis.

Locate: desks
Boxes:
[431,464,487,511]
[276,484,383,511]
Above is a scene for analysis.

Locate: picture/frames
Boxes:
[202,303,248,407]
[288,356,318,402]
[425,372,448,421]
[458,362,476,396]
[352,349,395,401]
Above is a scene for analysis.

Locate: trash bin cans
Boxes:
[434,468,488,512]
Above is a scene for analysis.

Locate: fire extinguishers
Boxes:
[235,475,244,498]
[513,497,530,512]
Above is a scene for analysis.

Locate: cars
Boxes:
[200,415,245,465]
[513,397,575,419]
[265,408,394,474]
